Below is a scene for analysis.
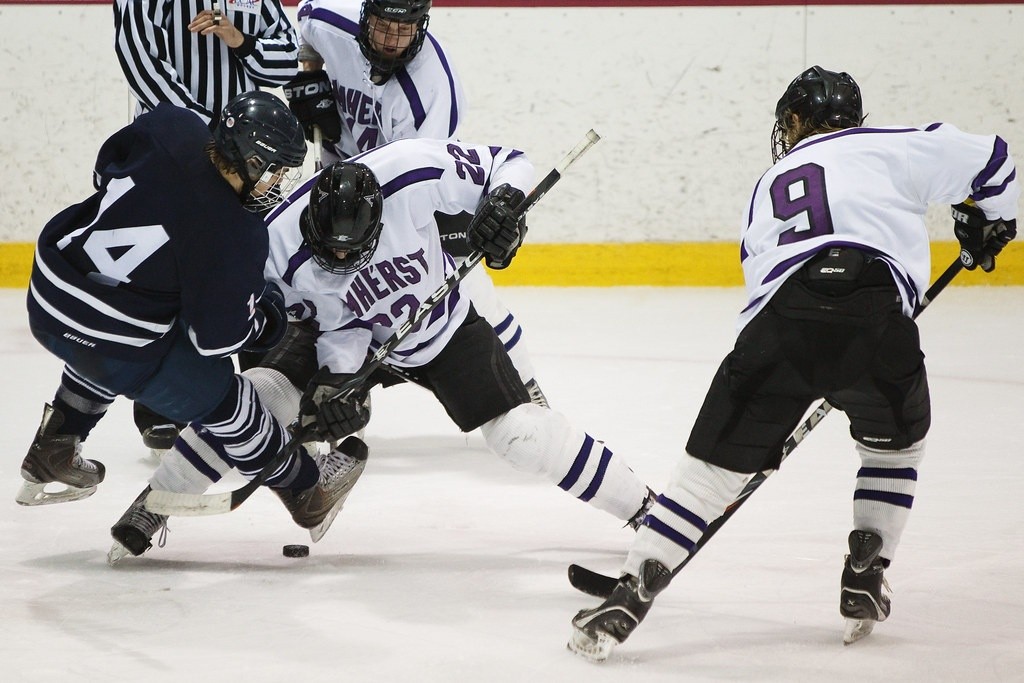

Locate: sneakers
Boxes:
[140,423,179,461]
[525,377,549,410]
[839,530,892,644]
[268,436,369,543]
[566,559,673,663]
[104,483,171,566]
[17,402,107,507]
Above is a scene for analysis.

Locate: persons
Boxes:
[14,0,661,564]
[568,64,1017,664]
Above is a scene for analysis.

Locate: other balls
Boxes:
[283,545,310,558]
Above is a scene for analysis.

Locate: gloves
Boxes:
[951,198,1017,272]
[465,183,528,269]
[245,280,287,356]
[282,71,341,151]
[296,366,372,443]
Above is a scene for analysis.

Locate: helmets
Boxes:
[774,65,863,128]
[218,90,308,184]
[309,160,383,248]
[363,0,432,33]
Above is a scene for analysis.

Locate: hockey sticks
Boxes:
[566,217,1015,598]
[144,127,604,518]
